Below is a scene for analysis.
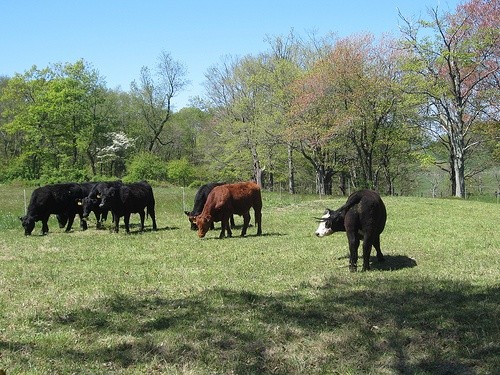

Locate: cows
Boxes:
[184,181,263,239]
[313,189,387,273]
[18,179,158,237]
